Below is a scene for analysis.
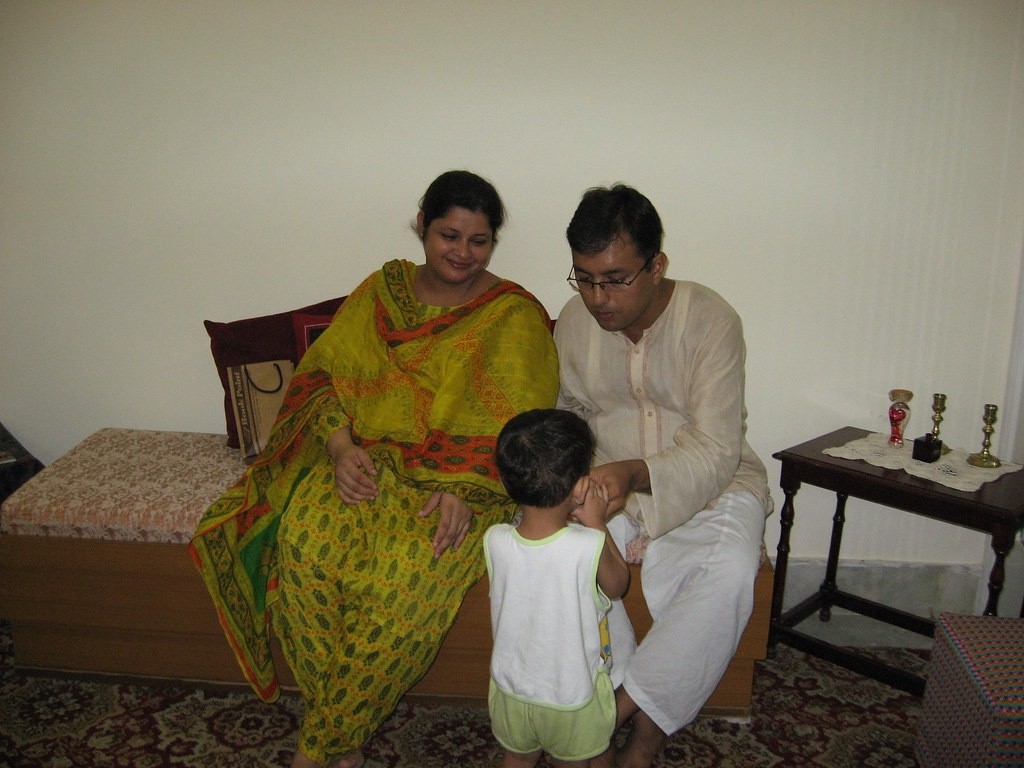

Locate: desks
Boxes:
[765,426,1024,703]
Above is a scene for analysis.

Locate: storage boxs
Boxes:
[912,436,942,464]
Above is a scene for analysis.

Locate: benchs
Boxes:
[0,427,777,717]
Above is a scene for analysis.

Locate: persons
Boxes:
[552,184,773,768]
[273,170,558,766]
[480,410,629,767]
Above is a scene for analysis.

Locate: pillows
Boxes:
[291,310,334,363]
[202,294,349,446]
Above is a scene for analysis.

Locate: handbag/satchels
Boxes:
[226,359,295,465]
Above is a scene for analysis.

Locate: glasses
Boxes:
[567,254,652,296]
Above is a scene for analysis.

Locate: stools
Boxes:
[913,611,1024,768]
